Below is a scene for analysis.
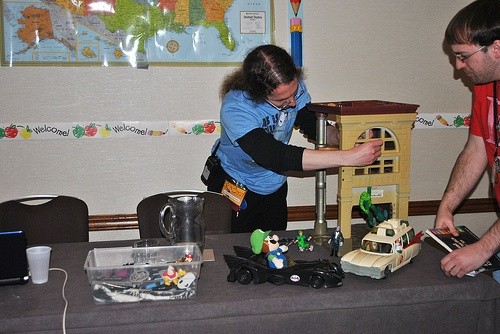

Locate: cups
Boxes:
[26,245,52,285]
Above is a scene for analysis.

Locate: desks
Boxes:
[0,230,500,334]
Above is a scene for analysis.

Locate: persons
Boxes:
[201,44,383,232]
[434,0,500,277]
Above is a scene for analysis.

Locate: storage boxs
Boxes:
[83,243,204,304]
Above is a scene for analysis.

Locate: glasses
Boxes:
[267,81,304,111]
[450,46,485,63]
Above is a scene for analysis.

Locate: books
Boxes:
[425,225,500,276]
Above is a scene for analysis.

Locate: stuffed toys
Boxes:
[250,229,289,269]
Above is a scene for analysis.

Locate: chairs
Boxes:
[136,190,232,239]
[0,195,89,244]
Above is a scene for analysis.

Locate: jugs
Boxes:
[159,196,205,252]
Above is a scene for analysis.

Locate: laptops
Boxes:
[0,232,29,285]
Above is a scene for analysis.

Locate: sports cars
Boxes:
[224,244,345,289]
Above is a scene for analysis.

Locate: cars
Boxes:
[339,217,422,281]
